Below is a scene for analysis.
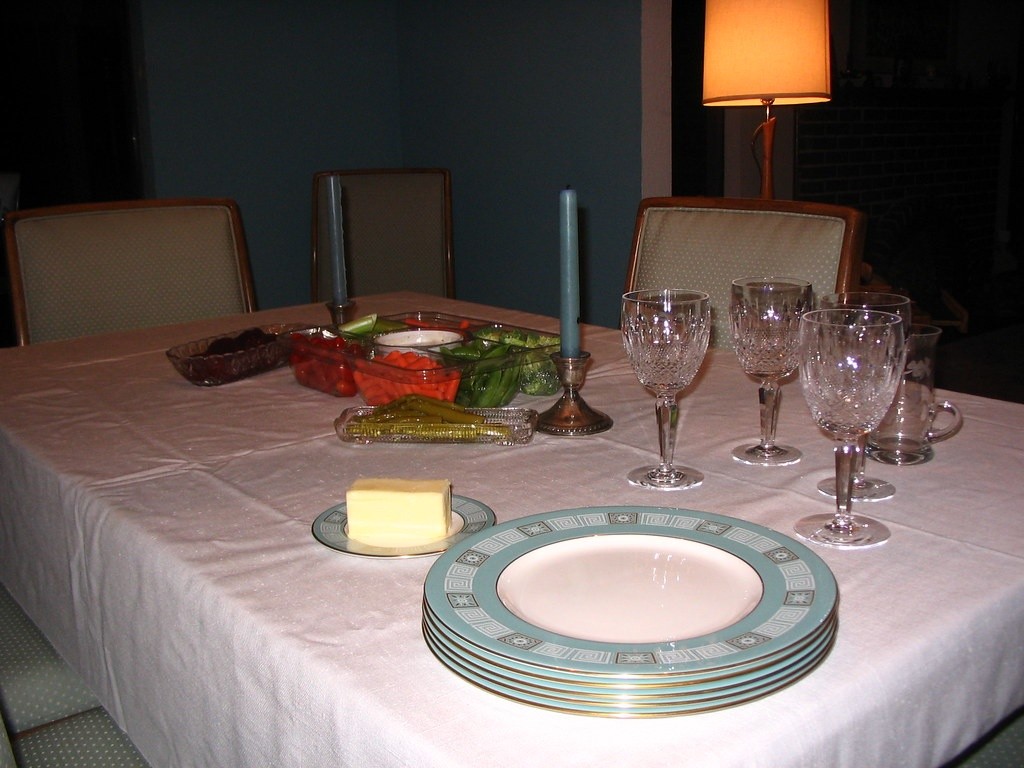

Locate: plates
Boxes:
[422,507,839,715]
[333,406,539,446]
[311,493,495,560]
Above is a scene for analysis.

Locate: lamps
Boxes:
[701,0,833,197]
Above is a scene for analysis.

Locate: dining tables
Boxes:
[0,289,1024,768]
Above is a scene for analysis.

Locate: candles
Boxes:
[325,170,346,306]
[558,185,581,357]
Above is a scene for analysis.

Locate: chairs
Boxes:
[5,195,257,350]
[0,583,150,768]
[620,196,862,350]
[309,166,454,306]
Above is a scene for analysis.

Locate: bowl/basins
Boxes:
[164,321,318,387]
[276,311,562,406]
[372,330,464,354]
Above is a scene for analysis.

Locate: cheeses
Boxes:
[345,477,451,540]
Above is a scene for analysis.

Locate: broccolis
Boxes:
[472,326,565,396]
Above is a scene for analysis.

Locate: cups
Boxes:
[864,322,961,465]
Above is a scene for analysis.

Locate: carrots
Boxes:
[404,317,469,335]
[352,351,460,406]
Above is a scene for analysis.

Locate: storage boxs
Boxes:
[275,311,560,408]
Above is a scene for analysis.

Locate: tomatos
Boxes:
[288,333,364,396]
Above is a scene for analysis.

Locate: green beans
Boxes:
[441,341,522,407]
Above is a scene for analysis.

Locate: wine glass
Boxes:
[797,309,904,550]
[817,291,911,502]
[729,276,815,465]
[620,288,711,489]
